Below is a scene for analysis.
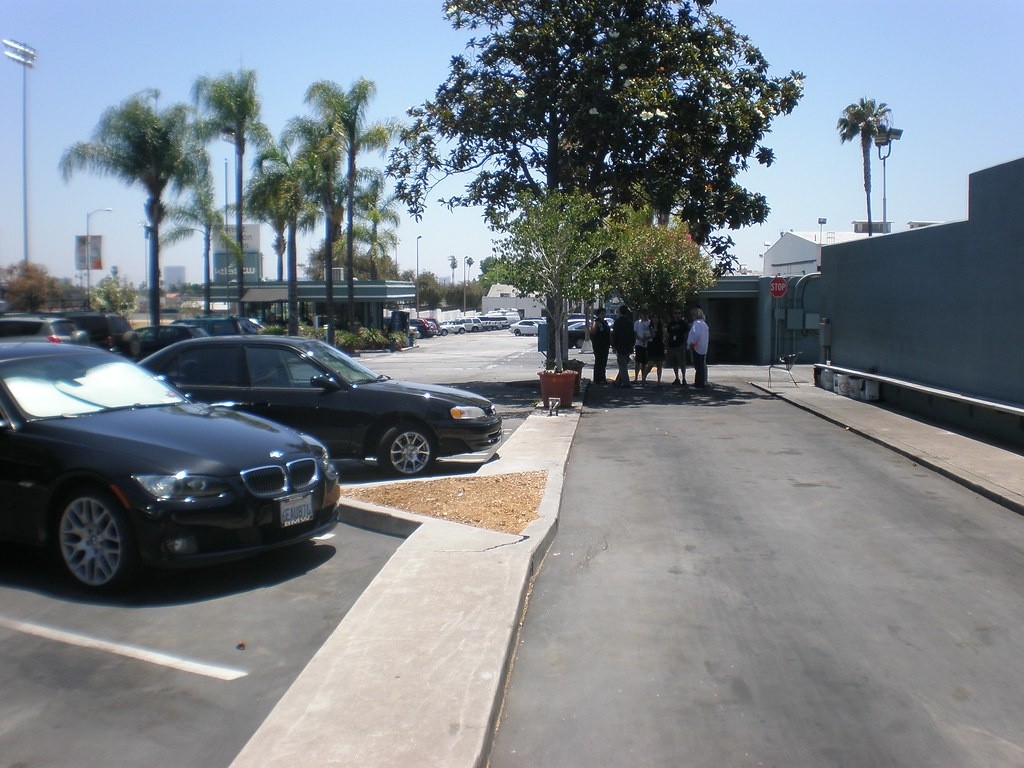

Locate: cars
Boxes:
[1,343,342,594]
[509,318,615,350]
[137,335,501,479]
[131,316,266,357]
[409,317,516,339]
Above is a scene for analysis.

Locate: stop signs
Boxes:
[770,277,788,297]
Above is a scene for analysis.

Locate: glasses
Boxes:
[642,313,649,315]
[674,312,681,314]
[653,317,658,318]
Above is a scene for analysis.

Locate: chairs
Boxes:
[768,352,803,387]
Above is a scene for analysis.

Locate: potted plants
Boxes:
[477,181,720,407]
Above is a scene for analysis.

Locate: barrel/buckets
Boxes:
[821,369,879,401]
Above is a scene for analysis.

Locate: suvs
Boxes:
[3,310,140,361]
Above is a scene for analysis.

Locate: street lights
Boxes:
[818,217,827,244]
[86,208,112,309]
[224,157,228,316]
[875,124,909,237]
[136,218,151,287]
[463,256,468,316]
[416,235,422,318]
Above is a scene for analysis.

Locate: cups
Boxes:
[826,360,831,366]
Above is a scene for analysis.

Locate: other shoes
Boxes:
[682,379,687,384]
[672,379,680,384]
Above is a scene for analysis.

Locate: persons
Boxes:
[590,305,709,391]
